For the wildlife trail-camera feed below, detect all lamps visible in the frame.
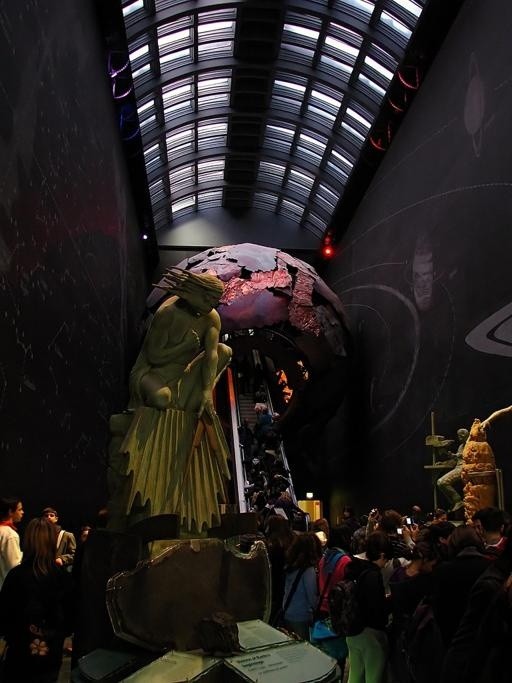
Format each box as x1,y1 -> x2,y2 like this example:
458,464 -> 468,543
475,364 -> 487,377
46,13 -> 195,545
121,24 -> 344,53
321,235 -> 334,257
368,123 -> 391,153
396,65 -> 419,90
107,49 -> 129,79
121,121 -> 139,141
112,80 -> 133,100
387,91 -> 407,114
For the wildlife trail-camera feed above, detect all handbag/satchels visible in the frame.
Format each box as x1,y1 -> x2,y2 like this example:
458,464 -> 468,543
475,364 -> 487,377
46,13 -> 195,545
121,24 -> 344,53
311,614 -> 340,640
272,608 -> 285,626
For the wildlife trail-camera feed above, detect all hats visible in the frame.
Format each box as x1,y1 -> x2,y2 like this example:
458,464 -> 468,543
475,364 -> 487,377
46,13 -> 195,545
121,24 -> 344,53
42,507 -> 57,516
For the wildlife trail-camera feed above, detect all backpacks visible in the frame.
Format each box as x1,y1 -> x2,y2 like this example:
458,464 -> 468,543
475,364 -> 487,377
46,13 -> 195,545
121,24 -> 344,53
328,560 -> 375,636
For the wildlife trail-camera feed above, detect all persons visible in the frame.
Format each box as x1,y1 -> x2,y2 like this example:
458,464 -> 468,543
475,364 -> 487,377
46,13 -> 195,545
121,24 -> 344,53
1,490 -> 133,681
124,264 -> 234,418
434,427 -> 468,516
250,473 -> 512,682
232,367 -> 294,486
